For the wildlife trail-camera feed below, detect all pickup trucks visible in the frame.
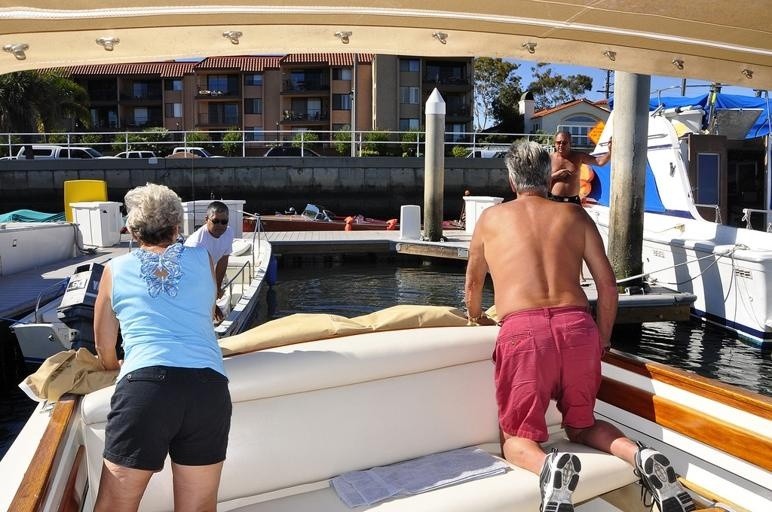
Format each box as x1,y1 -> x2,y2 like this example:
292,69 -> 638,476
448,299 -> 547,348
16,145 -> 121,160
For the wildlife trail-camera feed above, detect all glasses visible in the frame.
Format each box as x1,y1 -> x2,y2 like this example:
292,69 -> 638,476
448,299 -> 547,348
207,218 -> 228,225
554,140 -> 569,145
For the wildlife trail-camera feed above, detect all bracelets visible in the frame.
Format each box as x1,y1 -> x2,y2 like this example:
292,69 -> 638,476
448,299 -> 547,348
467,311 -> 487,323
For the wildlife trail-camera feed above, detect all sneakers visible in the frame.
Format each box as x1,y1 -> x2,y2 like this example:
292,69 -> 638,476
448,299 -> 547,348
539,447 -> 582,512
633,440 -> 696,512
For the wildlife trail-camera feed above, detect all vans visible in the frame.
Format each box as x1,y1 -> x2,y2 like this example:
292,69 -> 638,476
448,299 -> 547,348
173,146 -> 227,158
464,145 -> 511,159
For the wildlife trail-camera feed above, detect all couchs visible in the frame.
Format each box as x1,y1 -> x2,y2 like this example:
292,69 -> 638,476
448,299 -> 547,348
85,323 -> 646,511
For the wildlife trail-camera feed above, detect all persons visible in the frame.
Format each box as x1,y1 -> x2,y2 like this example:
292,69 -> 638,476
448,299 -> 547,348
464,137 -> 700,511
549,130 -> 613,208
182,201 -> 233,321
91,181 -> 233,511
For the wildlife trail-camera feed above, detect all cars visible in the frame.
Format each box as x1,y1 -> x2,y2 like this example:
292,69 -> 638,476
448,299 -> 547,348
264,146 -> 320,157
0,156 -> 17,160
113,150 -> 156,159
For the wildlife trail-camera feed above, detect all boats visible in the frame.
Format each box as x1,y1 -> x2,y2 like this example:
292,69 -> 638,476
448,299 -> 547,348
0,1 -> 772,512
576,77 -> 772,356
12,213 -> 277,367
242,204 -> 465,231
